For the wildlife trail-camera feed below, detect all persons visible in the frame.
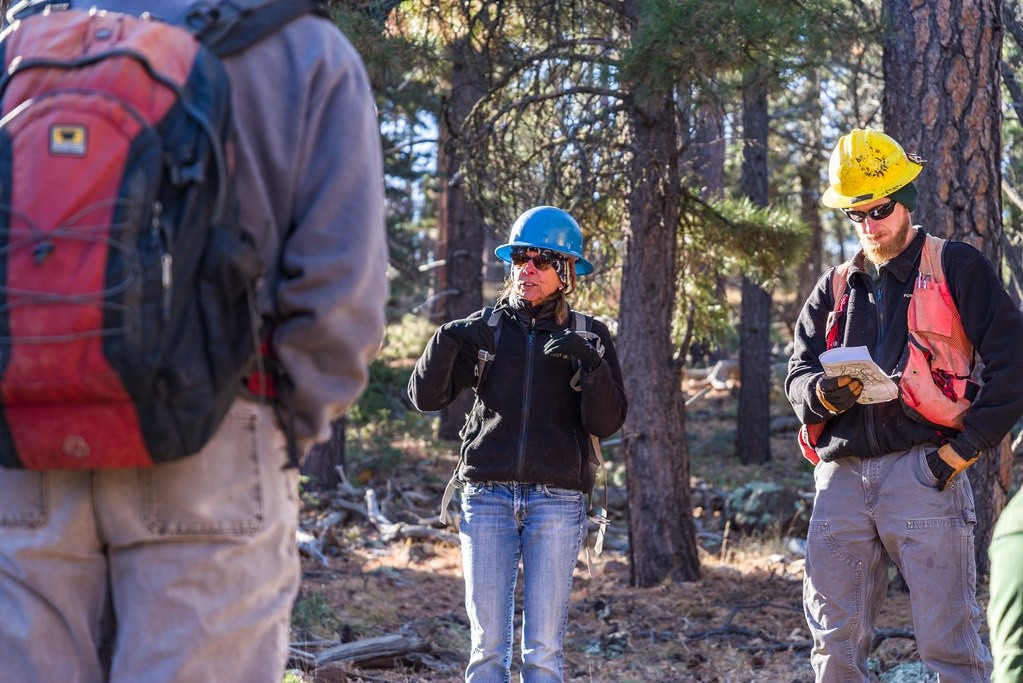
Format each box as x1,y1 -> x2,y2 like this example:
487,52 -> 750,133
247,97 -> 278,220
0,0 -> 383,683
408,206 -> 628,682
787,127 -> 1023,682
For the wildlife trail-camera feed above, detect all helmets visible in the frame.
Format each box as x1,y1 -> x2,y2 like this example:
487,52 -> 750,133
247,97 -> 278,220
822,127 -> 923,208
494,206 -> 593,275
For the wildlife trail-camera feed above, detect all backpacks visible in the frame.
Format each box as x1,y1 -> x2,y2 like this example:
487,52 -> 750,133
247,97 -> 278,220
0,0 -> 337,470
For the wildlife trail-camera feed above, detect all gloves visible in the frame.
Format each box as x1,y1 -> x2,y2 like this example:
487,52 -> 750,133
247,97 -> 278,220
544,327 -> 602,372
926,437 -> 982,492
441,317 -> 496,355
816,372 -> 864,416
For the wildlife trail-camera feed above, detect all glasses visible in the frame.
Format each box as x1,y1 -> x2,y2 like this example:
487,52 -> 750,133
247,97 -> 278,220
846,199 -> 897,222
510,252 -> 568,271
